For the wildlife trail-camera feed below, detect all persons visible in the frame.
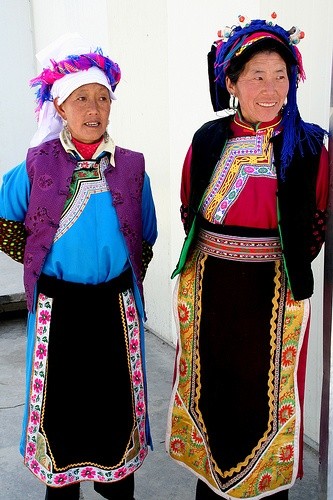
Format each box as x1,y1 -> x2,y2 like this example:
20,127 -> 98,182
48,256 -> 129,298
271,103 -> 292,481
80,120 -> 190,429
180,18 -> 328,500
0,52 -> 158,500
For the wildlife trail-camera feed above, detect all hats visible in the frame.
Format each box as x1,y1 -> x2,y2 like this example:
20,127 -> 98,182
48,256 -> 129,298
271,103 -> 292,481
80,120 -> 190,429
208,12 -> 329,180
29,47 -> 120,147
